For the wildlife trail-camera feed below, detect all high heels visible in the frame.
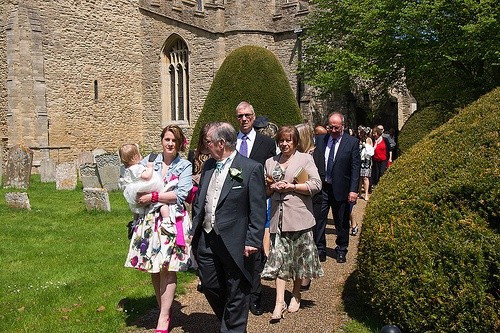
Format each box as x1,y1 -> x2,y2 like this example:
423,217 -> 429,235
154,307 -> 172,333
270,302 -> 288,320
288,299 -> 301,314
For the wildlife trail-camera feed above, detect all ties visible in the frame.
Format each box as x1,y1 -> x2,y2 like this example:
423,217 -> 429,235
239,136 -> 249,158
214,157 -> 232,178
326,138 -> 339,183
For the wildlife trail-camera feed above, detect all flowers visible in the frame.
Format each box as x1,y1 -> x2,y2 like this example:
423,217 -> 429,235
229,167 -> 244,182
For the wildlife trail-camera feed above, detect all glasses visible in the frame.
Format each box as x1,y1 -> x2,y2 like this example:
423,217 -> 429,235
206,140 -> 216,145
237,113 -> 253,119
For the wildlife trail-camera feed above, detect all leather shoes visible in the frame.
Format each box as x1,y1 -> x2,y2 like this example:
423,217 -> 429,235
251,304 -> 263,315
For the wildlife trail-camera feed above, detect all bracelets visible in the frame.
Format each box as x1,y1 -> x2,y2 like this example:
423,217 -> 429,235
293,184 -> 296,192
151,192 -> 158,203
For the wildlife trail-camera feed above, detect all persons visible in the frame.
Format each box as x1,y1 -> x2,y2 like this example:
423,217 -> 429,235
258,122 -> 281,155
295,124 -> 317,155
119,144 -> 177,234
124,124 -> 193,333
314,126 -> 327,134
189,123 -> 268,333
311,112 -> 361,264
343,122 -> 359,235
344,126 -> 396,200
188,122 -> 212,293
264,125 -> 324,320
235,102 -> 276,315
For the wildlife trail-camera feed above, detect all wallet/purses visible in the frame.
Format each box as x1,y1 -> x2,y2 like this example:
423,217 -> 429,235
293,168 -> 310,185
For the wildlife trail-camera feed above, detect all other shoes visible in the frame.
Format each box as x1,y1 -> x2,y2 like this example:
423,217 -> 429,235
337,253 -> 347,263
161,223 -> 177,236
350,223 -> 359,235
300,279 -> 312,291
318,252 -> 327,262
